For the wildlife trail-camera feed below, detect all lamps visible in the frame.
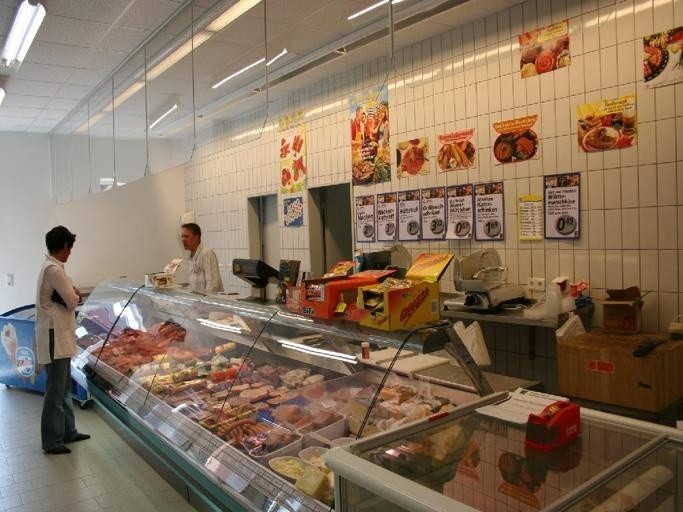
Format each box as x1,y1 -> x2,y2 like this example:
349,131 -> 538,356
0,0 -> 46,72
144,43 -> 290,132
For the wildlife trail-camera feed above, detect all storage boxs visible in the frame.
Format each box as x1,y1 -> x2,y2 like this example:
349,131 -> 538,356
300,273 -> 441,333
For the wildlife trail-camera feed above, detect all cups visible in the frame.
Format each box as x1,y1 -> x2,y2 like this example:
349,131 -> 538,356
623,115 -> 635,136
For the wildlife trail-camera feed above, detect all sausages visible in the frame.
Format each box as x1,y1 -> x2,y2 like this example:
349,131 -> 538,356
216,418 -> 272,448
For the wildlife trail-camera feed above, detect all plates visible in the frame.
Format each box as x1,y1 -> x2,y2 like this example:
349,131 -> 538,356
581,127 -> 620,150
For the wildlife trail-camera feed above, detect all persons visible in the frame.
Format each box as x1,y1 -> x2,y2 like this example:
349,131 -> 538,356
35,224 -> 91,454
180,222 -> 222,294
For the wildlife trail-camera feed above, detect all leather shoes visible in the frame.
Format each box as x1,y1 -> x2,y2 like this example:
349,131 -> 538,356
64,431 -> 92,443
43,445 -> 72,455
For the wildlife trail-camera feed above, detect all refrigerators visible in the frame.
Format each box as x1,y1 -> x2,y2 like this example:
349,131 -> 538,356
0,304 -> 90,408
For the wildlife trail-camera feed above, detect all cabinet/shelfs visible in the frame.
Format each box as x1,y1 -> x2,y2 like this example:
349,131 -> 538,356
0,280 -> 683,512
